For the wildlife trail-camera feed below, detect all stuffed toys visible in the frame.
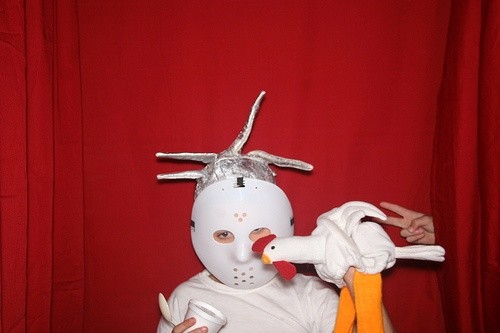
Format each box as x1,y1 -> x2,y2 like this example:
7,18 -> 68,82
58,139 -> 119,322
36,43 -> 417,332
252,201 -> 446,333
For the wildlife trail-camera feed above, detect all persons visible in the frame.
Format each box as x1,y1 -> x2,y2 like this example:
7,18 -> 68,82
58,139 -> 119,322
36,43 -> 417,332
156,91 -> 395,333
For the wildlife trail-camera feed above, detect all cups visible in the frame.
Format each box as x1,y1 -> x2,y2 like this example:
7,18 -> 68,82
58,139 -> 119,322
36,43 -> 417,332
184,299 -> 226,333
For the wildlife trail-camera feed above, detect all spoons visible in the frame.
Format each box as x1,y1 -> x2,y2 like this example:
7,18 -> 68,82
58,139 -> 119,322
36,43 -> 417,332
158,293 -> 179,327
